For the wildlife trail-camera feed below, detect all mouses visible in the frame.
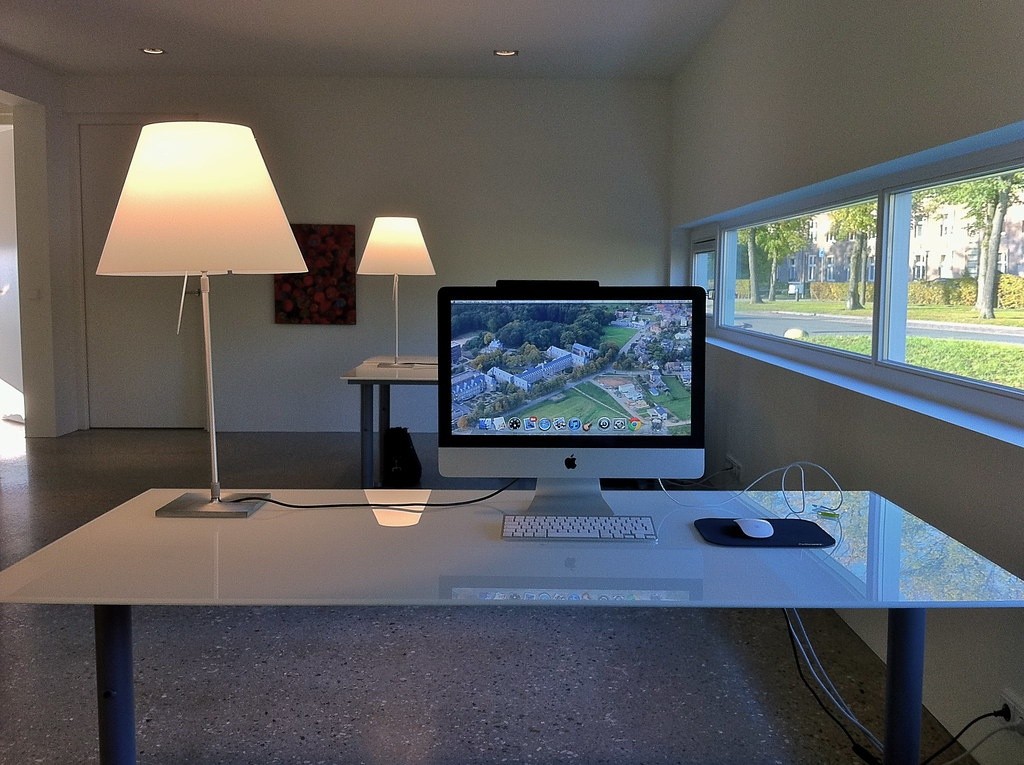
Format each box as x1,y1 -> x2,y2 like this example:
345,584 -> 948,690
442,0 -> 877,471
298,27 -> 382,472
733,519 -> 774,539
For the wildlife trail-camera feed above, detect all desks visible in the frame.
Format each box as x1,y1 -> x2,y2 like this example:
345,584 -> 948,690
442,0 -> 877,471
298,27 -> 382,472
3,478 -> 1024,765
339,353 -> 439,491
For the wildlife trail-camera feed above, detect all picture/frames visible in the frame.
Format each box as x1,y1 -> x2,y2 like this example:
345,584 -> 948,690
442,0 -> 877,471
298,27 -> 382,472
273,220 -> 359,327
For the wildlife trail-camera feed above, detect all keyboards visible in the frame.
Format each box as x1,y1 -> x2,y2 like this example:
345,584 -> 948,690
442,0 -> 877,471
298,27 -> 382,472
501,514 -> 658,543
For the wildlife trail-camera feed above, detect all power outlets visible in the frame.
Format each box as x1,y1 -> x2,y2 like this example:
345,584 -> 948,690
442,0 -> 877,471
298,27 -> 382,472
997,688 -> 1024,737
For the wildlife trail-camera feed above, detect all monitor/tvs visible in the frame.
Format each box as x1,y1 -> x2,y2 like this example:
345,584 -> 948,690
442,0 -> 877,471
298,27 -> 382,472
436,286 -> 707,476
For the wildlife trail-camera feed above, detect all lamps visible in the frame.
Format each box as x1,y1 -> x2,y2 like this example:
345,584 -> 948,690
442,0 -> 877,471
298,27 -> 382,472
355,214 -> 438,371
88,113 -> 313,520
